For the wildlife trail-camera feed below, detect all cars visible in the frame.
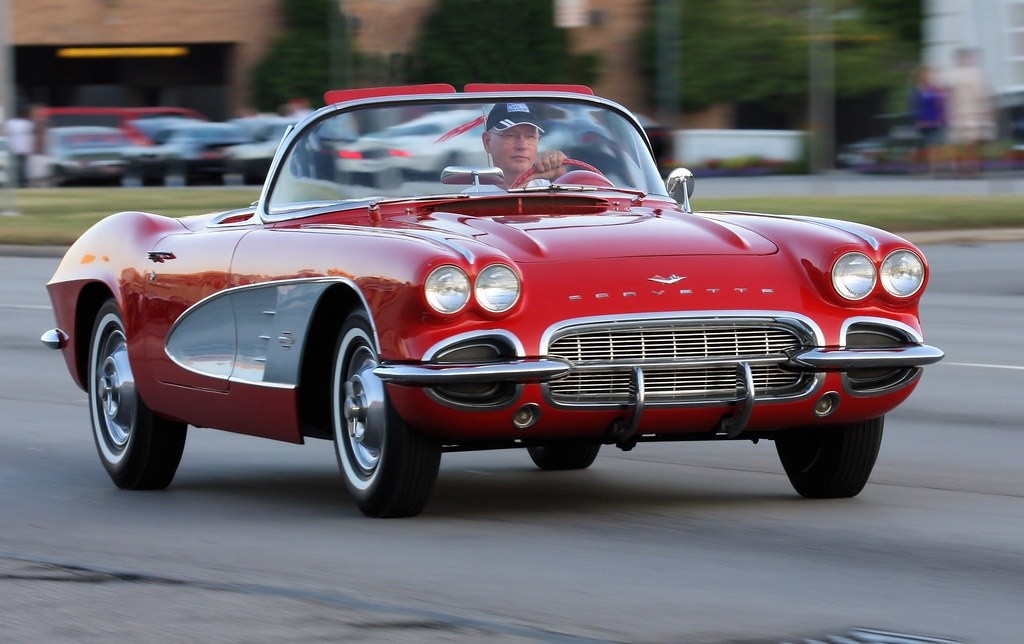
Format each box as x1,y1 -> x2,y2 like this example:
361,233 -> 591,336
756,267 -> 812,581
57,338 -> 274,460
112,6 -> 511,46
0,113 -> 671,189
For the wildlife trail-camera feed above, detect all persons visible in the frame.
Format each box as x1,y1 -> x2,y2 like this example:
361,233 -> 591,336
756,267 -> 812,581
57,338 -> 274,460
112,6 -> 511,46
3,112 -> 35,187
461,102 -> 569,193
912,46 -> 997,147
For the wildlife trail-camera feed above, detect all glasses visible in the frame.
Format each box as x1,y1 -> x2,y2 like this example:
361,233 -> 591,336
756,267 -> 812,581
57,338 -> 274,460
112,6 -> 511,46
487,130 -> 542,145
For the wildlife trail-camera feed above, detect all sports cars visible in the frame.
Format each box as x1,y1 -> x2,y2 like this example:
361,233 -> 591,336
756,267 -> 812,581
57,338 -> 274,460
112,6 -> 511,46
40,83 -> 945,520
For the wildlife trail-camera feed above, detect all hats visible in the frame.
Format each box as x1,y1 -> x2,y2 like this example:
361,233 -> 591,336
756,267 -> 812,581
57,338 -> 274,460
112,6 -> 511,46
486,102 -> 546,134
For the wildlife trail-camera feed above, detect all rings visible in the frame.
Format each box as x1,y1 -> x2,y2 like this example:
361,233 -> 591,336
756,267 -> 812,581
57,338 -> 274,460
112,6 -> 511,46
550,154 -> 557,158
